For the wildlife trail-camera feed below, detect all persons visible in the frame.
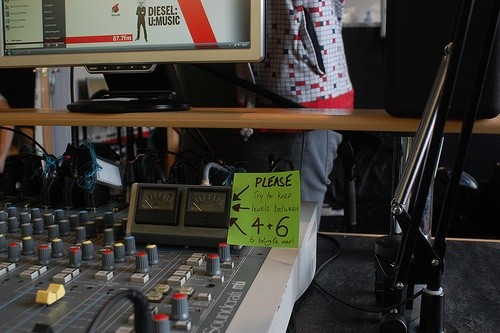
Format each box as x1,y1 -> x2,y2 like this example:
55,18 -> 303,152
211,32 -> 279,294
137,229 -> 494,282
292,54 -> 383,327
249,0 -> 354,231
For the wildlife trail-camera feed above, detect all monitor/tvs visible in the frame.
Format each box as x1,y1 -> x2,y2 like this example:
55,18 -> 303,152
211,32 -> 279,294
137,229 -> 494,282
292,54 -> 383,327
0,0 -> 268,114
